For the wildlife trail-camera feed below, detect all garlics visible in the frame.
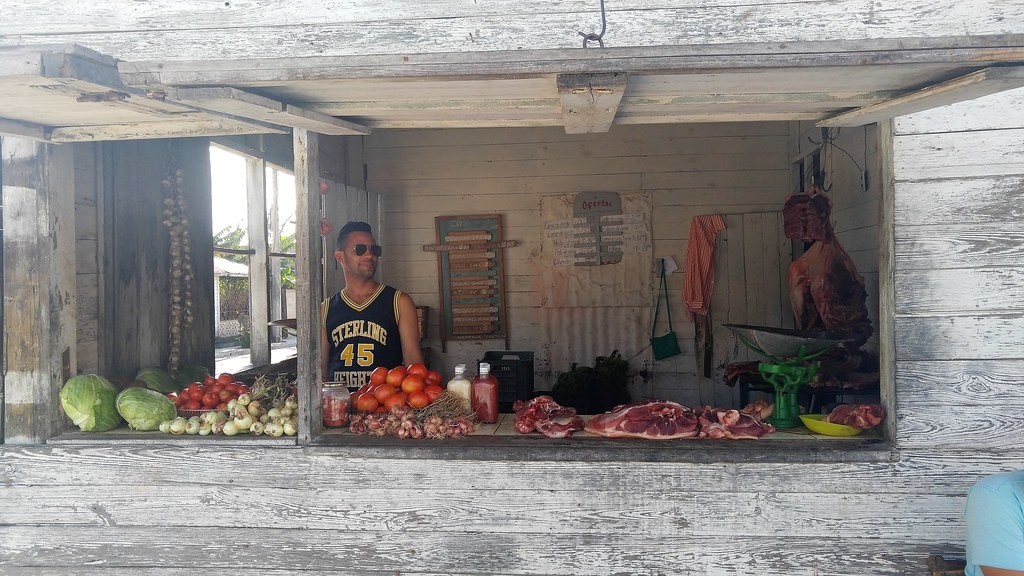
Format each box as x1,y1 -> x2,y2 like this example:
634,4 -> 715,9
161,169 -> 193,373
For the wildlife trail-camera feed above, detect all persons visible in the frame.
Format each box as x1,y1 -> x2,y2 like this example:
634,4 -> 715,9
320,221 -> 423,393
963,468 -> 1024,576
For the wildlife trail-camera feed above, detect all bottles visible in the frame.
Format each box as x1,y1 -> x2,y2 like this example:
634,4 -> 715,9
473,363 -> 498,424
448,364 -> 473,419
323,382 -> 350,429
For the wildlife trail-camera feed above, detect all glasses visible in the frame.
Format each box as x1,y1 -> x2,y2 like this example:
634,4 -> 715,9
339,244 -> 382,257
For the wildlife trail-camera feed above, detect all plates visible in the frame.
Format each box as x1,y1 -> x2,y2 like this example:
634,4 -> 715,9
800,414 -> 864,437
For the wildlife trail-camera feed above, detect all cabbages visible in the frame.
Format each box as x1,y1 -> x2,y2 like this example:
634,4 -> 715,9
59,364 -> 176,432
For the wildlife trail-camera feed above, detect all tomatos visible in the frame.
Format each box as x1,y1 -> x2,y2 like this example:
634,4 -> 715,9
166,372 -> 250,416
349,363 -> 442,413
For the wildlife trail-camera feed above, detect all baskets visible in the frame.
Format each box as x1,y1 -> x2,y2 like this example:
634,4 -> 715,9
175,408 -> 229,418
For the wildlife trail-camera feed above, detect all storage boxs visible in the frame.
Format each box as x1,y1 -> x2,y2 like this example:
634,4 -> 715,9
477,351 -> 535,412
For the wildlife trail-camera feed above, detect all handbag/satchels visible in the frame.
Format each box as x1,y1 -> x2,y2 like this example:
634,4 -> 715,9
649,331 -> 682,362
594,349 -> 628,384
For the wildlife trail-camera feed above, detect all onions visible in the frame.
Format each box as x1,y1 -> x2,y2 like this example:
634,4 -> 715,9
159,394 -> 299,438
346,405 -> 477,440
